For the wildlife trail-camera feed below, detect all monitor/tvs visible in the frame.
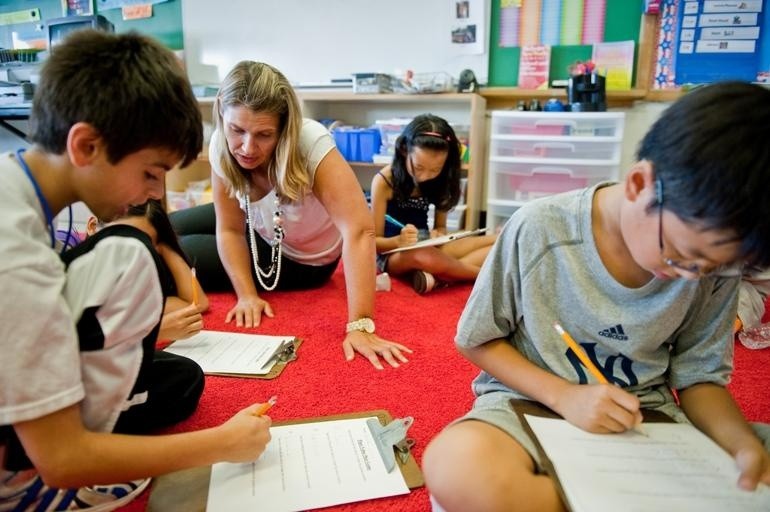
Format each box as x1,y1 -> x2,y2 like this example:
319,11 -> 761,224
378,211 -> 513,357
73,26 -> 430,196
47,14 -> 115,47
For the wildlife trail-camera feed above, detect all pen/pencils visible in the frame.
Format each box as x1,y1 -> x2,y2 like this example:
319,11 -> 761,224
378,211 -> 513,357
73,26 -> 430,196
551,319 -> 650,437
569,61 -> 599,103
384,214 -> 424,241
191,267 -> 198,308
254,396 -> 278,418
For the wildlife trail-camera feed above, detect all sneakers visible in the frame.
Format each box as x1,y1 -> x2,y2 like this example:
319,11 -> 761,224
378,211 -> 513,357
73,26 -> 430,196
0,474 -> 154,512
406,269 -> 437,294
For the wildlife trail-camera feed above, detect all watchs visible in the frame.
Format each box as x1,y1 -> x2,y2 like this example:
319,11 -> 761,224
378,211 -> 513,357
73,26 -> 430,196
345,316 -> 376,334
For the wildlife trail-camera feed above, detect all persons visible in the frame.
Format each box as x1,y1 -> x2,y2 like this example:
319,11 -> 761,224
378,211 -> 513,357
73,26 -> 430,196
166,60 -> 414,371
56,194 -> 212,345
0,25 -> 274,510
367,111 -> 497,298
414,79 -> 770,512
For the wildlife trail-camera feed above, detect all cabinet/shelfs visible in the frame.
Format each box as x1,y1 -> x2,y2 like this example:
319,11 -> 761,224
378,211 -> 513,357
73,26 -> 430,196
165,96 -> 217,213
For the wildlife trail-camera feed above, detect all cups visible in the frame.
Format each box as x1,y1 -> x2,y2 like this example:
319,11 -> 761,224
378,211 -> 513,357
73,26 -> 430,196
738,323 -> 770,350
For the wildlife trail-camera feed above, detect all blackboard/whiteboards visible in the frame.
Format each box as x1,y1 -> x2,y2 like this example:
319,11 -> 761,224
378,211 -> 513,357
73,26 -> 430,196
181,0 -> 492,88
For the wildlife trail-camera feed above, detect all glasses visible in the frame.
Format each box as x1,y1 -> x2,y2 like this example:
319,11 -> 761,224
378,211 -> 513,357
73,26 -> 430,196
641,156 -> 750,283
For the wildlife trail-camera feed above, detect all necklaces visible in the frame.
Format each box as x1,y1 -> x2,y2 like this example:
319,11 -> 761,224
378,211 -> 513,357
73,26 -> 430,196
243,186 -> 286,293
